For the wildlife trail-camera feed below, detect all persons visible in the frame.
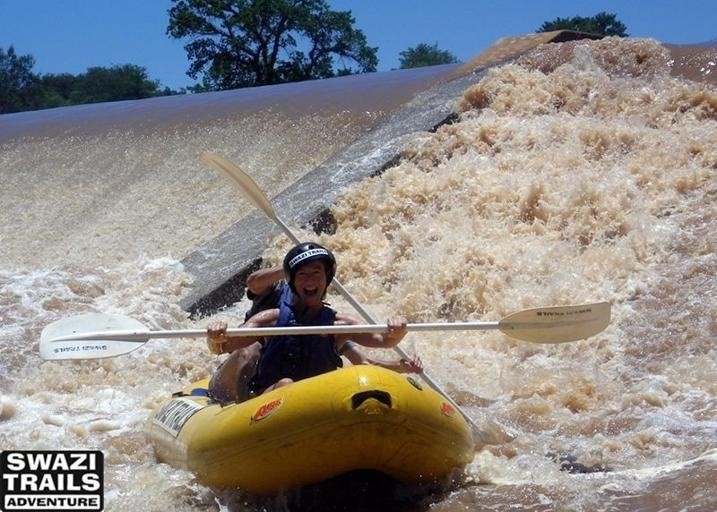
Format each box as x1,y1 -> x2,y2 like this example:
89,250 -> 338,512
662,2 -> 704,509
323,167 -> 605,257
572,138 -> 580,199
209,264 -> 424,402
206,242 -> 407,395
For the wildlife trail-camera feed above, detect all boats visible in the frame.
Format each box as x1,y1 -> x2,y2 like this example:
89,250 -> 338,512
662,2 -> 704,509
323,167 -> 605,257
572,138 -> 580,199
143,365 -> 478,496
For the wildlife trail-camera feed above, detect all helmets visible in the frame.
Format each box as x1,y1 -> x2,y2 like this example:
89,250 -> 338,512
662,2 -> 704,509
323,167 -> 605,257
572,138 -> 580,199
282,241 -> 337,293
